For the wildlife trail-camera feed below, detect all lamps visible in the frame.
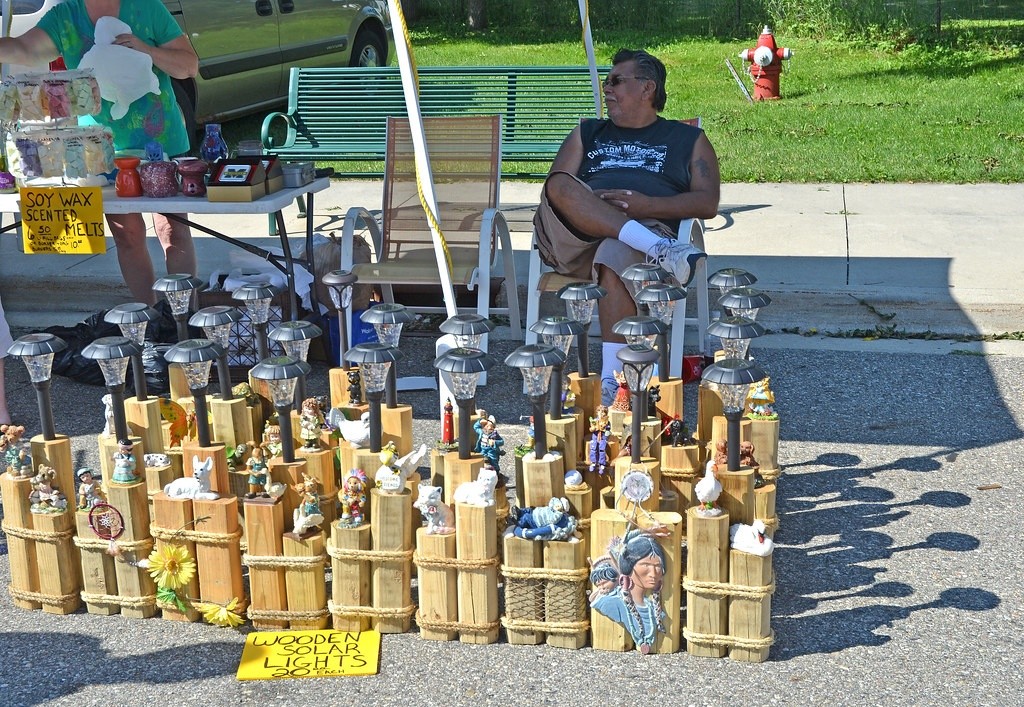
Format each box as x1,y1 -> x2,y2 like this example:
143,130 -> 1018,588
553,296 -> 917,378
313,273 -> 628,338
560,282 -> 607,376
616,344 -> 660,463
233,283 -> 280,361
718,288 -> 771,322
343,341 -> 402,453
267,321 -> 323,417
250,356 -> 311,463
635,284 -> 689,380
323,269 -> 359,371
707,268 -> 758,298
8,273 -> 243,447
702,358 -> 764,471
432,349 -> 498,460
503,344 -> 565,457
438,313 -> 495,418
622,264 -> 673,316
526,318 -> 587,417
706,316 -> 764,359
612,316 -> 669,419
359,305 -> 415,408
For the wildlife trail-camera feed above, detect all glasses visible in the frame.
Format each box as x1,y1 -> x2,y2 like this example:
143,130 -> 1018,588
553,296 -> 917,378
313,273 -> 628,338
602,75 -> 649,88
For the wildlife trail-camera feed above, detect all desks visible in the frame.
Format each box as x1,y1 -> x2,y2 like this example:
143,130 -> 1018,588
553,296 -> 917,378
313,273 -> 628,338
0,160 -> 328,363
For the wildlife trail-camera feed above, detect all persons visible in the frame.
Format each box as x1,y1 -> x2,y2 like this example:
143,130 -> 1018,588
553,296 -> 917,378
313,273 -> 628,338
78,466 -> 103,509
112,438 -> 139,481
262,426 -> 284,457
561,376 -> 576,414
512,498 -> 571,539
0,0 -> 202,308
587,407 -> 615,474
532,48 -> 721,405
300,397 -> 322,448
473,414 -> 506,476
525,418 -> 538,448
0,302 -> 13,433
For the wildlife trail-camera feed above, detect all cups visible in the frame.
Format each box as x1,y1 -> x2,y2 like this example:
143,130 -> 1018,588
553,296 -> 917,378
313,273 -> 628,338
237,141 -> 263,156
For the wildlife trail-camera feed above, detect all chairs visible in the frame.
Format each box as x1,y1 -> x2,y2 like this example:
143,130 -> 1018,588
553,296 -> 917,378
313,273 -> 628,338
339,116 -> 522,392
523,115 -> 702,394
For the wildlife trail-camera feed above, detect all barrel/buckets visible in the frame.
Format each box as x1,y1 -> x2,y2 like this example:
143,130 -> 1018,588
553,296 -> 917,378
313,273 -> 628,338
326,300 -> 381,366
683,355 -> 704,383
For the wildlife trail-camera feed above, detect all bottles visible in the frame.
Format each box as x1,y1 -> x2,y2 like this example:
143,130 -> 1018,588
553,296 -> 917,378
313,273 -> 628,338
113,158 -> 142,197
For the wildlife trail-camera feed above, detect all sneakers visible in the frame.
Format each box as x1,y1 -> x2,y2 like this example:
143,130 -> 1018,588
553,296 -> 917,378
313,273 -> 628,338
601,376 -> 618,407
646,237 -> 707,287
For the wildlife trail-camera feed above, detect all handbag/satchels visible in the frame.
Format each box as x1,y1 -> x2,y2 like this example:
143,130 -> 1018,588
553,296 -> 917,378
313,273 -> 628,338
324,301 -> 383,366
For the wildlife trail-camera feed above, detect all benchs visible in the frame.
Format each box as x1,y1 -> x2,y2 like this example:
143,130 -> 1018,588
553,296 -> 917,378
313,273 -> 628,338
262,67 -> 610,237
